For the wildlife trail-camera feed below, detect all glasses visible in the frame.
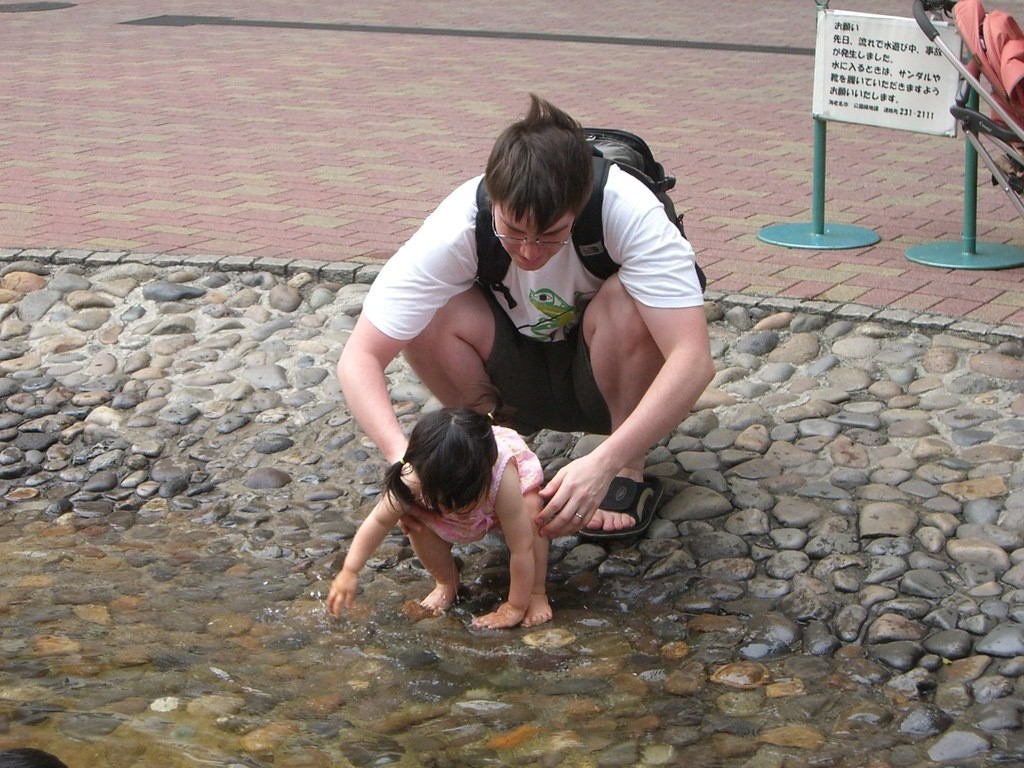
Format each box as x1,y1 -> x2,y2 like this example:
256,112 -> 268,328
492,204 -> 575,247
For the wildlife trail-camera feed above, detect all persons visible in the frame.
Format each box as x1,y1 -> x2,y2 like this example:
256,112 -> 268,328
325,379 -> 553,629
337,90 -> 716,538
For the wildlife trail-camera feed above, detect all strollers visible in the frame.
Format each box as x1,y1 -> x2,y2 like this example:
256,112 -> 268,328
911,0 -> 1024,218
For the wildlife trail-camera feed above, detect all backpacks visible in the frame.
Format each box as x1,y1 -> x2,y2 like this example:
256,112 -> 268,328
476,128 -> 707,310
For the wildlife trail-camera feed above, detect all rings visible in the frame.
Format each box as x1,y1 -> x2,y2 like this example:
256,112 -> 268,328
575,513 -> 583,519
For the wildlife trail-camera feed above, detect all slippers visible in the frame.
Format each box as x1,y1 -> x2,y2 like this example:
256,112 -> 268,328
578,474 -> 664,540
